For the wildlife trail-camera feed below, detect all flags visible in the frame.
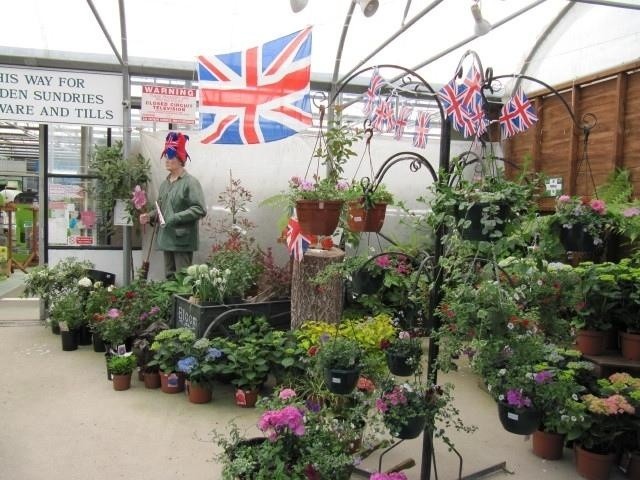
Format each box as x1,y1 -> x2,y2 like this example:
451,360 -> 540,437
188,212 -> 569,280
283,217 -> 314,264
193,24 -> 315,145
359,65 -> 540,151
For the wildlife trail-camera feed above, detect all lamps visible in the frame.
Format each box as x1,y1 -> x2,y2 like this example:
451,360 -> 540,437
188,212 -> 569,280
290,0 -> 308,13
352,0 -> 379,17
471,4 -> 491,36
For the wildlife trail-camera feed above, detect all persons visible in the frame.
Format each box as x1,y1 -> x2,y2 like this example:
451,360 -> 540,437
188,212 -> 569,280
139,131 -> 208,281
3,229 -> 9,244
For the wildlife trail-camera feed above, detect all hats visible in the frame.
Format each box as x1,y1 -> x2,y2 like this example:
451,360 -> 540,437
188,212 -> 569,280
161,133 -> 190,162
